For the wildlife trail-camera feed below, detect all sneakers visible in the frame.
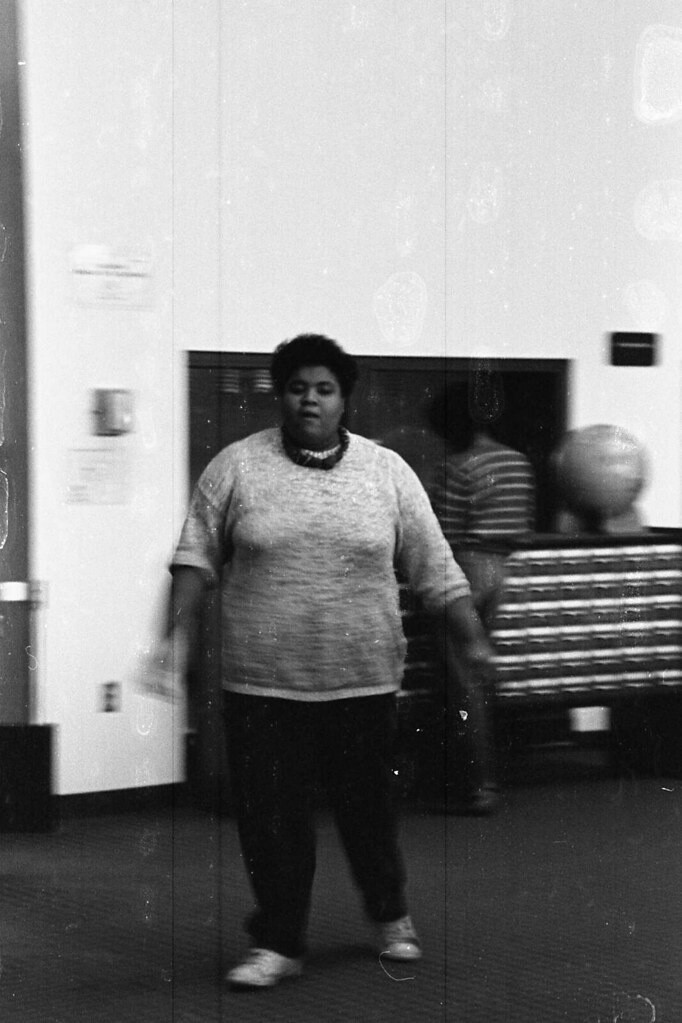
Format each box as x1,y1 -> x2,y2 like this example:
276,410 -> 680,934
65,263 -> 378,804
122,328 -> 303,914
225,948 -> 304,986
368,915 -> 422,982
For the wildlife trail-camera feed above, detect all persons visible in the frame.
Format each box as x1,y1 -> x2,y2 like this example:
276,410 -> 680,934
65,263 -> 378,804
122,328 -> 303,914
428,391 -> 540,816
144,334 -> 496,990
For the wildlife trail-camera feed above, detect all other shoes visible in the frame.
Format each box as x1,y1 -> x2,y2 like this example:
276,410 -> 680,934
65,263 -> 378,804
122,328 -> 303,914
430,787 -> 500,813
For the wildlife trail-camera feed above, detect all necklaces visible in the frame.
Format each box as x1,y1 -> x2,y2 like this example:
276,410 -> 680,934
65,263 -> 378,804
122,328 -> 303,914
281,422 -> 350,470
